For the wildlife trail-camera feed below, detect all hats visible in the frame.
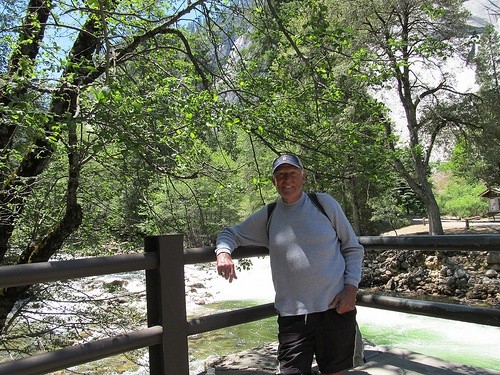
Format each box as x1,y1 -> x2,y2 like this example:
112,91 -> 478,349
272,154 -> 304,172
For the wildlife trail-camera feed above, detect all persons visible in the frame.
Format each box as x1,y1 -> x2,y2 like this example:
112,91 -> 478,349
214,155 -> 364,375
